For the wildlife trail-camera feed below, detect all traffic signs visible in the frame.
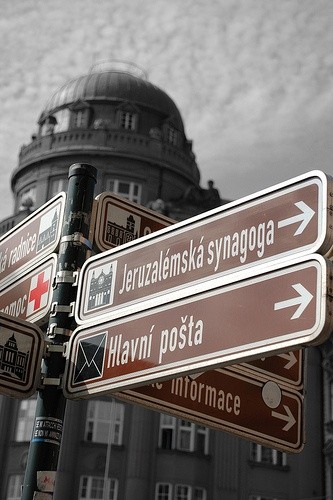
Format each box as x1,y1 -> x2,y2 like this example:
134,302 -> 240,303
73,170 -> 333,333
0,252 -> 59,326
108,361 -> 306,456
0,311 -> 46,403
0,190 -> 67,292
61,251 -> 331,407
220,340 -> 306,391
88,191 -> 180,255
